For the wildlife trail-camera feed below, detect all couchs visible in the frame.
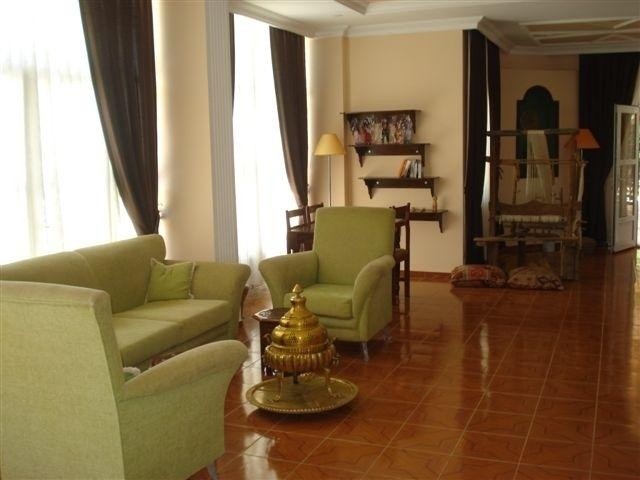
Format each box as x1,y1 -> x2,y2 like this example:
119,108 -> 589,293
0,231 -> 252,365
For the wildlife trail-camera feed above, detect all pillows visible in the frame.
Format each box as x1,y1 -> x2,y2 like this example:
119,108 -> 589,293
144,256 -> 196,301
449,264 -> 564,292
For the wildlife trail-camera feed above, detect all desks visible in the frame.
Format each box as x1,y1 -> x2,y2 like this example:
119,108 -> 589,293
252,307 -> 307,383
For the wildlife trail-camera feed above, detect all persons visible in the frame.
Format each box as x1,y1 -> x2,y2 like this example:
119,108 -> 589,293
350,113 -> 413,145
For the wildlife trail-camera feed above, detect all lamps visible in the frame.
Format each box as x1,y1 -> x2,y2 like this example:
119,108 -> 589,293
566,129 -> 602,164
312,132 -> 346,209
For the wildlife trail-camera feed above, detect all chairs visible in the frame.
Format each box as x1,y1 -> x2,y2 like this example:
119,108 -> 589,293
256,205 -> 398,363
286,202 -> 412,305
0,281 -> 250,480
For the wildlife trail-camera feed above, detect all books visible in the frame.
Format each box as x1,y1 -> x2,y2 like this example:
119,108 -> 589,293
400,159 -> 422,179
